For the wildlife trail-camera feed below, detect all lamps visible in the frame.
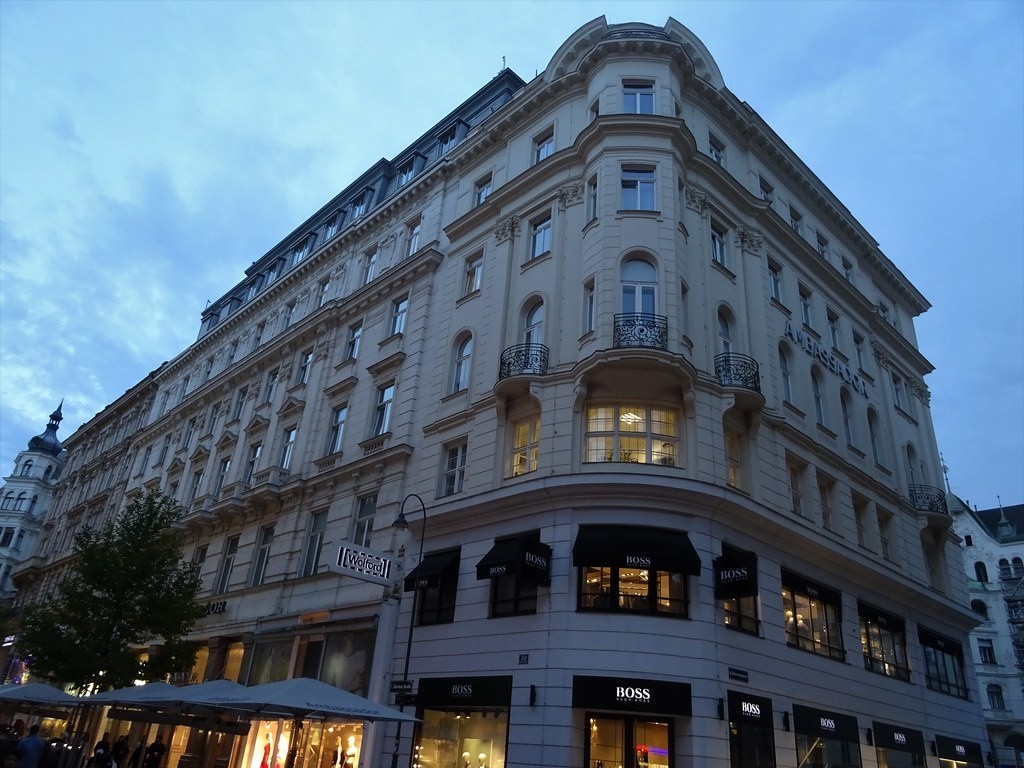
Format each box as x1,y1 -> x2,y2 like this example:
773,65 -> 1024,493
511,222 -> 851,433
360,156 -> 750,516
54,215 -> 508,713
619,407 -> 641,424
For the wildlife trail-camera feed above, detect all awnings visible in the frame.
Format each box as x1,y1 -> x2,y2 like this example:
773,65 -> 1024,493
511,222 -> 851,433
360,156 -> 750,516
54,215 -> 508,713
476,538 -> 550,579
404,547 -> 460,591
572,524 -> 701,576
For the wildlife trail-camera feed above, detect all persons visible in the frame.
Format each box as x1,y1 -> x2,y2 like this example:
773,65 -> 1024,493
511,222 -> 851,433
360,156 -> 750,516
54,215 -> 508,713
259,733 -> 284,768
327,637 -> 367,697
259,647 -> 286,684
331,736 -> 358,768
0,724 -> 166,768
786,610 -> 809,649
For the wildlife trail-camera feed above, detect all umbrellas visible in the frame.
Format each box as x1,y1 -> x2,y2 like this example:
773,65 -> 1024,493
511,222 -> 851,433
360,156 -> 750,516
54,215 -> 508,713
0,677 -> 426,723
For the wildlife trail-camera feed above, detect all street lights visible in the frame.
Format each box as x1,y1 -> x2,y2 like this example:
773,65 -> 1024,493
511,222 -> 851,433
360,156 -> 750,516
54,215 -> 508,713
389,493 -> 427,768
